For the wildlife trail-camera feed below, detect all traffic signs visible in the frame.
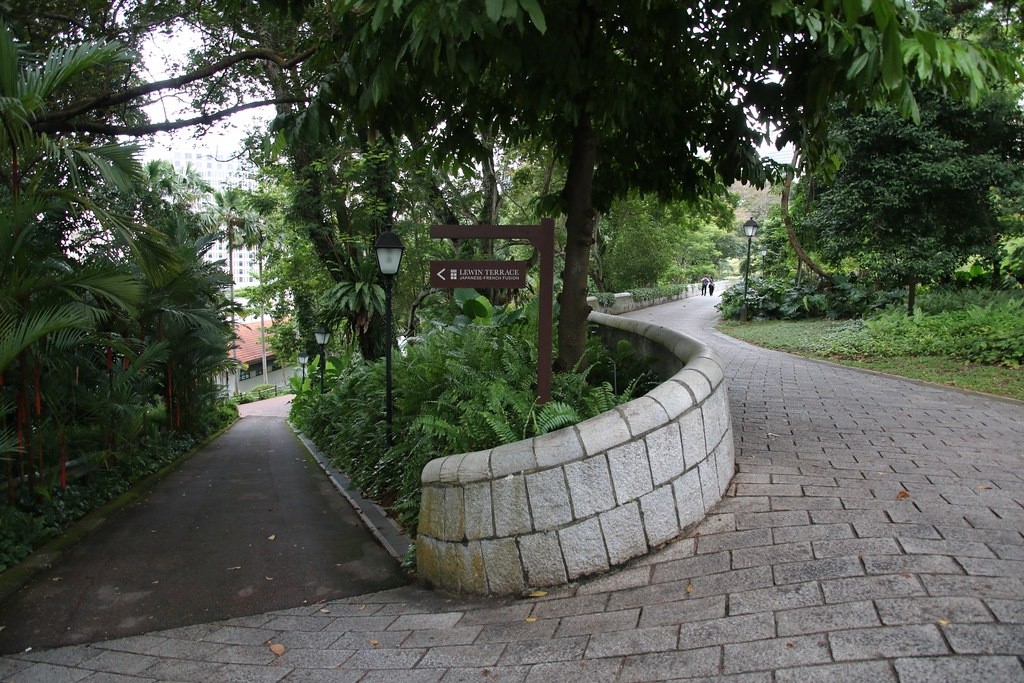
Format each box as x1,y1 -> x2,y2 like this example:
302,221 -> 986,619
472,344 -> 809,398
428,258 -> 527,290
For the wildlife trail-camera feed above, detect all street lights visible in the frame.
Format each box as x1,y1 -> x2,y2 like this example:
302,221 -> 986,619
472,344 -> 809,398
740,217 -> 758,323
761,244 -> 768,278
372,222 -> 407,453
312,323 -> 331,394
297,350 -> 310,385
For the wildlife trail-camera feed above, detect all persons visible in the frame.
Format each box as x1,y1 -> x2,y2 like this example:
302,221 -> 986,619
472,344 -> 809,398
700,276 -> 709,296
708,277 -> 715,296
